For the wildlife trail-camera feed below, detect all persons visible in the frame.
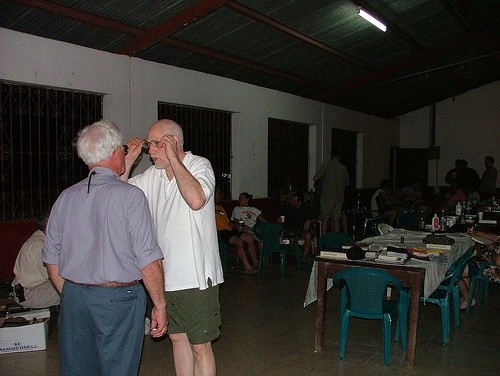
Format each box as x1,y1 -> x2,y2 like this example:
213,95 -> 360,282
214,188 -> 259,274
445,156 -> 497,204
41,120 -> 168,376
276,193 -> 320,264
371,179 -> 417,226
313,149 -> 350,235
11,216 -> 61,337
447,228 -> 500,309
231,192 -> 274,266
119,119 -> 224,376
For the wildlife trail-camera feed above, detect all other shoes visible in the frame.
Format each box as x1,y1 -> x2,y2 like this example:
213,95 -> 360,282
144,318 -> 152,336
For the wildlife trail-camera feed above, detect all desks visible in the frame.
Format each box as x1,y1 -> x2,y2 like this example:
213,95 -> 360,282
312,228 -> 481,369
409,201 -> 500,236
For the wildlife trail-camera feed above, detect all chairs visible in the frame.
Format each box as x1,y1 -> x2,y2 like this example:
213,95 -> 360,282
216,209 -> 500,366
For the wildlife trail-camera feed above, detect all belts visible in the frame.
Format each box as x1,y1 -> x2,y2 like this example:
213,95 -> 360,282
74,280 -> 139,287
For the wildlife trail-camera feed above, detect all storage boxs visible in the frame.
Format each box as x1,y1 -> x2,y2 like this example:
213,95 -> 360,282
0,318 -> 50,355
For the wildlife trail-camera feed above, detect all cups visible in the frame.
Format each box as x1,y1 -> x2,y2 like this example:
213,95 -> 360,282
281,216 -> 285,222
478,212 -> 483,221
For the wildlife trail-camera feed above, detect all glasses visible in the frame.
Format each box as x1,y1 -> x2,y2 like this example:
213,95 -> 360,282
144,140 -> 160,148
113,145 -> 129,156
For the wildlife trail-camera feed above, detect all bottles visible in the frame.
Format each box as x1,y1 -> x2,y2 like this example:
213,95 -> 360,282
449,218 -> 454,228
432,214 -> 439,231
462,202 -> 466,209
441,210 -> 447,231
461,216 -> 465,226
456,202 -> 461,215
419,210 -> 425,230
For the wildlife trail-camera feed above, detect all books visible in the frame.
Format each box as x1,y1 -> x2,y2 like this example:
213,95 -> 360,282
342,243 -> 369,250
426,234 -> 451,251
379,245 -> 409,264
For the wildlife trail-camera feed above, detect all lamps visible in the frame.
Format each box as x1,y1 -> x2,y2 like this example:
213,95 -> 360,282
355,6 -> 388,32
